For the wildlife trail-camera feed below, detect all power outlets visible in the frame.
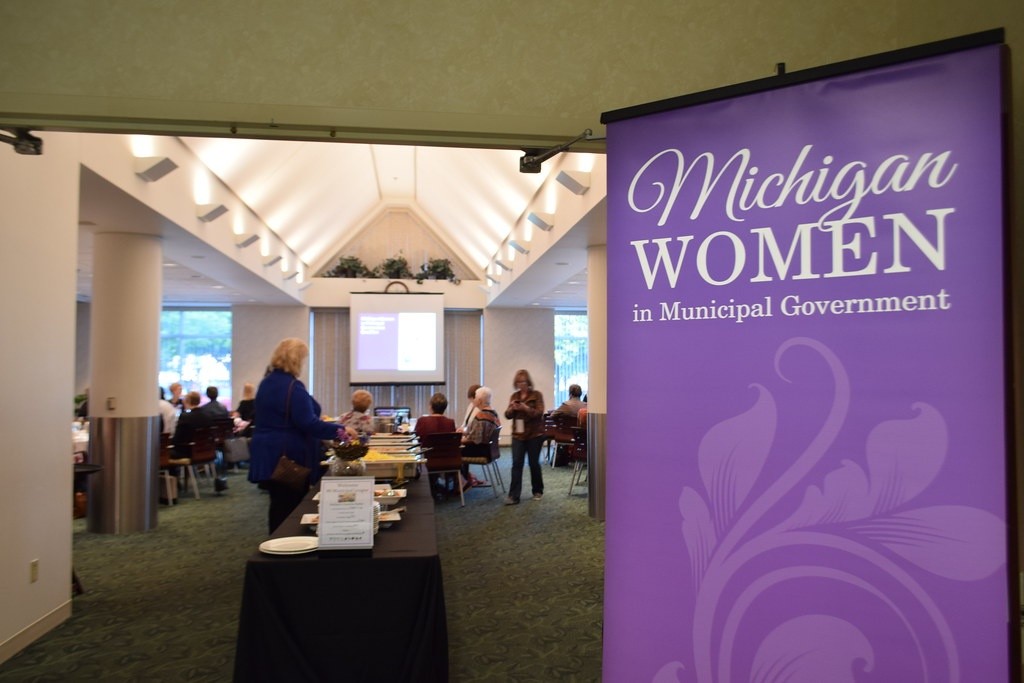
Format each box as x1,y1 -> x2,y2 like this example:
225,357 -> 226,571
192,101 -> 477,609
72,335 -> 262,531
29,559 -> 39,584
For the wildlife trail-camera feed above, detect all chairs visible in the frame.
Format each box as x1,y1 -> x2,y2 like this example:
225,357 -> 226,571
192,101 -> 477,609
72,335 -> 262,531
422,432 -> 465,507
462,426 -> 506,499
158,417 -> 234,506
545,409 -> 587,498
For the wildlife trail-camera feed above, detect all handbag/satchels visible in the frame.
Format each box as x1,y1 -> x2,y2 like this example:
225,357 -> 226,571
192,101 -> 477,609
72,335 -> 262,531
215,477 -> 229,491
271,456 -> 311,491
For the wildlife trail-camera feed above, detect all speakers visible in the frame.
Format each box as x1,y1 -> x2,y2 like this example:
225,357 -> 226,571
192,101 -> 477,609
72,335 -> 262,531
283,271 -> 299,280
261,255 -> 282,266
235,233 -> 260,248
197,203 -> 228,223
135,156 -> 178,183
487,273 -> 500,283
299,283 -> 314,290
479,284 -> 490,294
556,169 -> 591,195
495,259 -> 513,271
509,240 -> 530,254
528,211 -> 554,231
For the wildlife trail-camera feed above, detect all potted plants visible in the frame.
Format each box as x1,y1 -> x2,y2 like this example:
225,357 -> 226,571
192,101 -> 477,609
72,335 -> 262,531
416,257 -> 462,286
323,254 -> 374,278
371,249 -> 416,278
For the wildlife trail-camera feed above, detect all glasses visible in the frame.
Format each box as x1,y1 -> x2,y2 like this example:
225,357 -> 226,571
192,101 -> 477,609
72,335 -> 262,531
517,382 -> 526,385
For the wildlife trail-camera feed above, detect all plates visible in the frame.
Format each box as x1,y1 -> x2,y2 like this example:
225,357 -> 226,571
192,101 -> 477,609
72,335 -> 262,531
259,536 -> 320,554
300,512 -> 401,530
312,489 -> 408,504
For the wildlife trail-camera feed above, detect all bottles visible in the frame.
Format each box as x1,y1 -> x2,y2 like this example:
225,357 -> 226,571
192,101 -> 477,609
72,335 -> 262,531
391,411 -> 410,433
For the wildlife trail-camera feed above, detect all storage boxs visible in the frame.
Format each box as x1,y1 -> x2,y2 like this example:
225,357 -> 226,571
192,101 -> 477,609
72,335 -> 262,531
369,432 -> 416,438
368,446 -> 422,454
366,438 -> 420,446
322,454 -> 427,477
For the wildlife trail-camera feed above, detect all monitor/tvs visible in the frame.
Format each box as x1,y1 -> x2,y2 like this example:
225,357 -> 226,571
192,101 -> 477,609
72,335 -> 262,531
373,407 -> 410,431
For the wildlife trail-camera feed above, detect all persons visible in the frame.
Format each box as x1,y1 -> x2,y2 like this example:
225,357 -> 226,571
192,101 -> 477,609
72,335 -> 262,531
246,337 -> 359,536
455,384 -> 501,495
550,384 -> 587,427
229,382 -> 256,438
414,392 -> 457,502
159,383 -> 229,460
503,369 -> 544,505
335,389 -> 376,433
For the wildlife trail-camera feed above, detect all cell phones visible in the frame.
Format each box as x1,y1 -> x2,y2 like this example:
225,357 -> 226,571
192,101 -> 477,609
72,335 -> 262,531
514,400 -> 520,404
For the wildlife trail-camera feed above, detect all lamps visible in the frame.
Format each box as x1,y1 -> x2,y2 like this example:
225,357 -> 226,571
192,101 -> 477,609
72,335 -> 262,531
479,285 -> 491,294
196,203 -> 229,223
134,155 -> 178,183
555,169 -> 592,196
235,234 -> 260,248
298,282 -> 312,291
263,255 -> 283,267
508,239 -> 531,256
527,211 -> 554,231
485,274 -> 501,284
495,259 -> 513,272
282,271 -> 300,280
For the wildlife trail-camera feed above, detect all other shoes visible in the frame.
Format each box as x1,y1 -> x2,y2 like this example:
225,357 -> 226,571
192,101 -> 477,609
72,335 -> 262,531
532,493 -> 544,500
451,489 -> 459,495
505,498 -> 517,504
464,476 -> 483,492
436,493 -> 442,501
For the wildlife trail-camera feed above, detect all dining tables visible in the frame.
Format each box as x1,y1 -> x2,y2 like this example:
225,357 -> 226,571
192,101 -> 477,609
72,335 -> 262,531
237,431 -> 449,683
